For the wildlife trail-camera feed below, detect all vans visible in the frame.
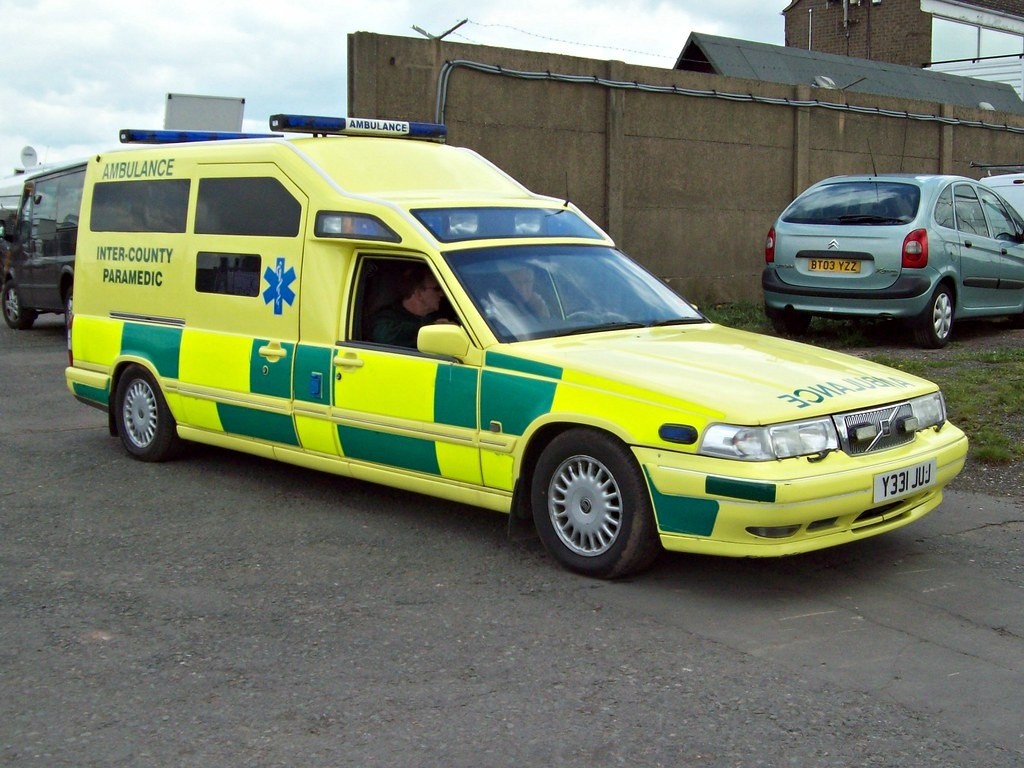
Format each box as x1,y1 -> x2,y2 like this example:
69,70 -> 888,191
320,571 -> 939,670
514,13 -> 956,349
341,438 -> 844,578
0,159 -> 89,332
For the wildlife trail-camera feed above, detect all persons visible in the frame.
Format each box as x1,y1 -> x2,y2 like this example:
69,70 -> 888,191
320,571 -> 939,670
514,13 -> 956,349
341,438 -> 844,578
0,214 -> 18,243
369,267 -> 459,353
488,260 -> 554,342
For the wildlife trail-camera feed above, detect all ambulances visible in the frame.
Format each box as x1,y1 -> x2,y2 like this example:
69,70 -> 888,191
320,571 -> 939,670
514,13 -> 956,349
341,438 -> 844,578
64,112 -> 970,581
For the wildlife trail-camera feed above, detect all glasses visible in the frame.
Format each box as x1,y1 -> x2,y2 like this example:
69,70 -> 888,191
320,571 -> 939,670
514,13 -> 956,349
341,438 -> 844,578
420,285 -> 443,292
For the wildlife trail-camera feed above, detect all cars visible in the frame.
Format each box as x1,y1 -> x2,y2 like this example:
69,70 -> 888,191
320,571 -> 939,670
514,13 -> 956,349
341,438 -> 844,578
761,173 -> 1024,349
978,173 -> 1023,229
